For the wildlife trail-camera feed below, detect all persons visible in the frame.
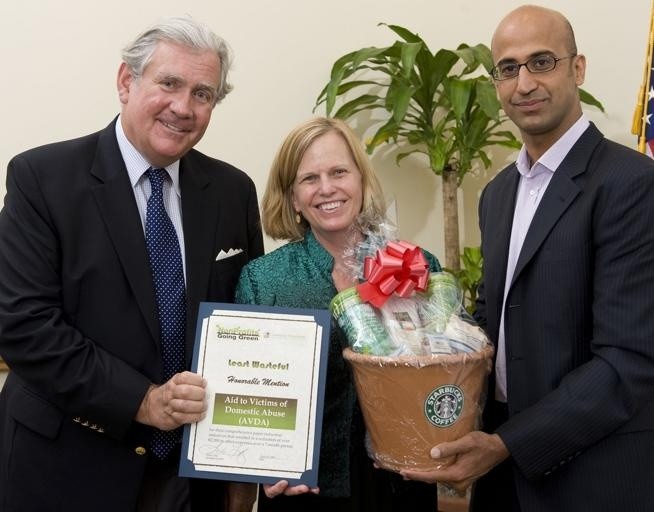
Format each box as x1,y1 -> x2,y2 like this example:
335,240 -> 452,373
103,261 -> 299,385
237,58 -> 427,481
0,17 -> 266,512
235,117 -> 442,512
401,2 -> 654,512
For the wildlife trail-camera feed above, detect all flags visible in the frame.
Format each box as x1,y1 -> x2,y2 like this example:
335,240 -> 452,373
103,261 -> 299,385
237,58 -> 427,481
632,6 -> 654,158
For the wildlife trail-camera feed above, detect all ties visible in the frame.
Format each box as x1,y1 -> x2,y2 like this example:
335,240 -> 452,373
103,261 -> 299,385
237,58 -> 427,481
146,167 -> 186,461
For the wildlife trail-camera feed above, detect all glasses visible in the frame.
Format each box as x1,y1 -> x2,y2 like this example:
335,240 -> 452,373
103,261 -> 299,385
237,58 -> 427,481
489,55 -> 574,82
